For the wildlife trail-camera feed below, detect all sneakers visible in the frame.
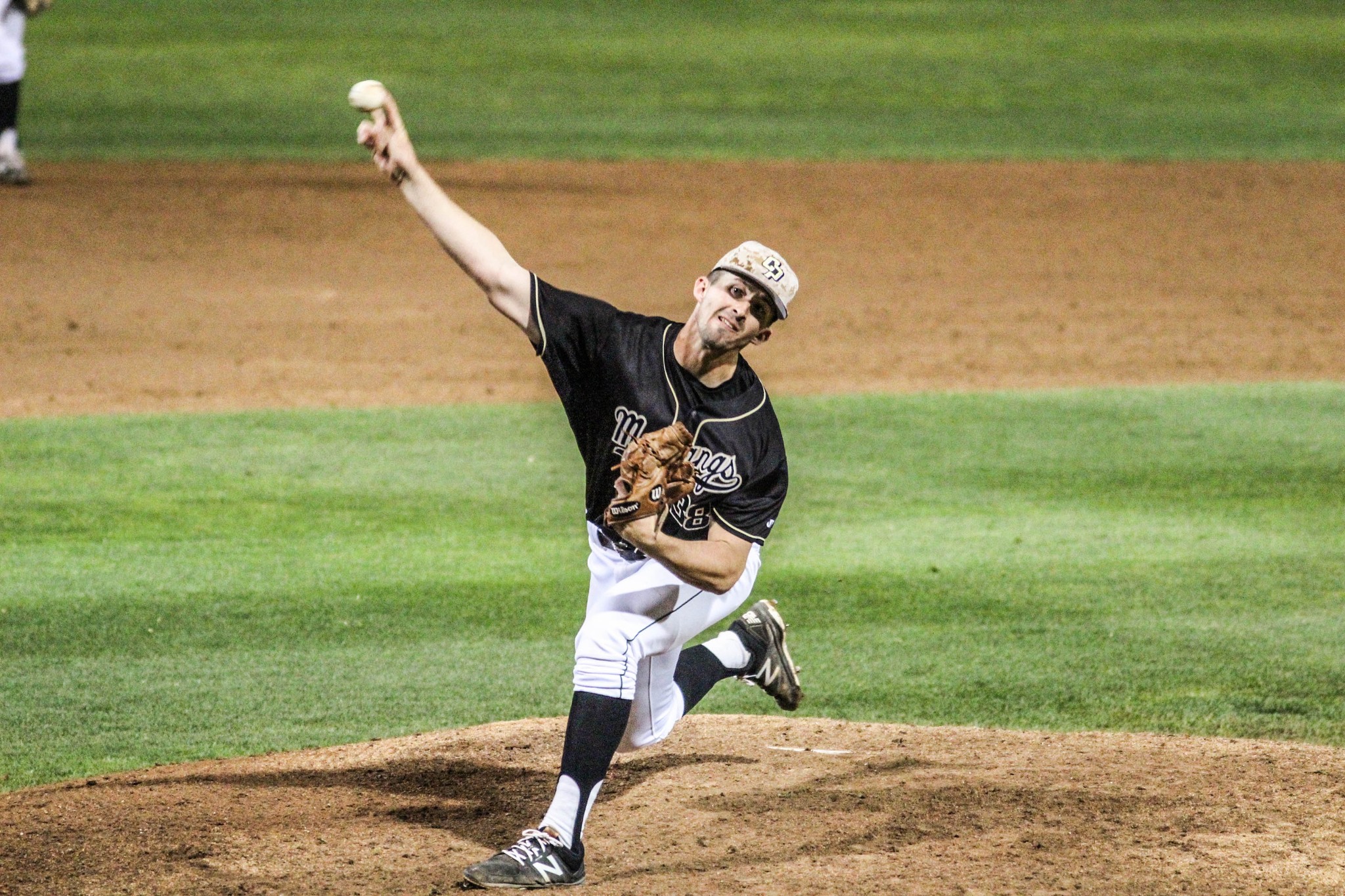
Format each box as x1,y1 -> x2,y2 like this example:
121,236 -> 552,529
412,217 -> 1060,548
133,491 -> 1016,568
732,599 -> 803,710
464,826 -> 587,890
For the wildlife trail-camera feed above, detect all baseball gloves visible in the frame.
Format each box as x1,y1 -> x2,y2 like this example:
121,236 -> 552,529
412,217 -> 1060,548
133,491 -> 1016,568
602,421 -> 698,529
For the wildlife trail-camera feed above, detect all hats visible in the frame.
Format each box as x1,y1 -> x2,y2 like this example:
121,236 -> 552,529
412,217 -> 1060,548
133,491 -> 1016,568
711,239 -> 798,321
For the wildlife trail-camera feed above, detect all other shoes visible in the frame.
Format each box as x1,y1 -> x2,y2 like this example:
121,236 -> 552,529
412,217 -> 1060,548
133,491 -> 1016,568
0,150 -> 29,186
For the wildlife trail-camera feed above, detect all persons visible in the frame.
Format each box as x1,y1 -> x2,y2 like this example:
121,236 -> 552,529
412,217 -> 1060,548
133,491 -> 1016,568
0,1 -> 55,188
345,92 -> 808,892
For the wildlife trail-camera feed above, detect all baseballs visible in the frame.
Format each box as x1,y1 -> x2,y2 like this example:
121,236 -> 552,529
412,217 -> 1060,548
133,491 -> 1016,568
347,78 -> 387,113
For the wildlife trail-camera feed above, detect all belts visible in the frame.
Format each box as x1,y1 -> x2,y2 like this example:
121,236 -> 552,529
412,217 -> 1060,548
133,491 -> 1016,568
598,531 -> 648,562
